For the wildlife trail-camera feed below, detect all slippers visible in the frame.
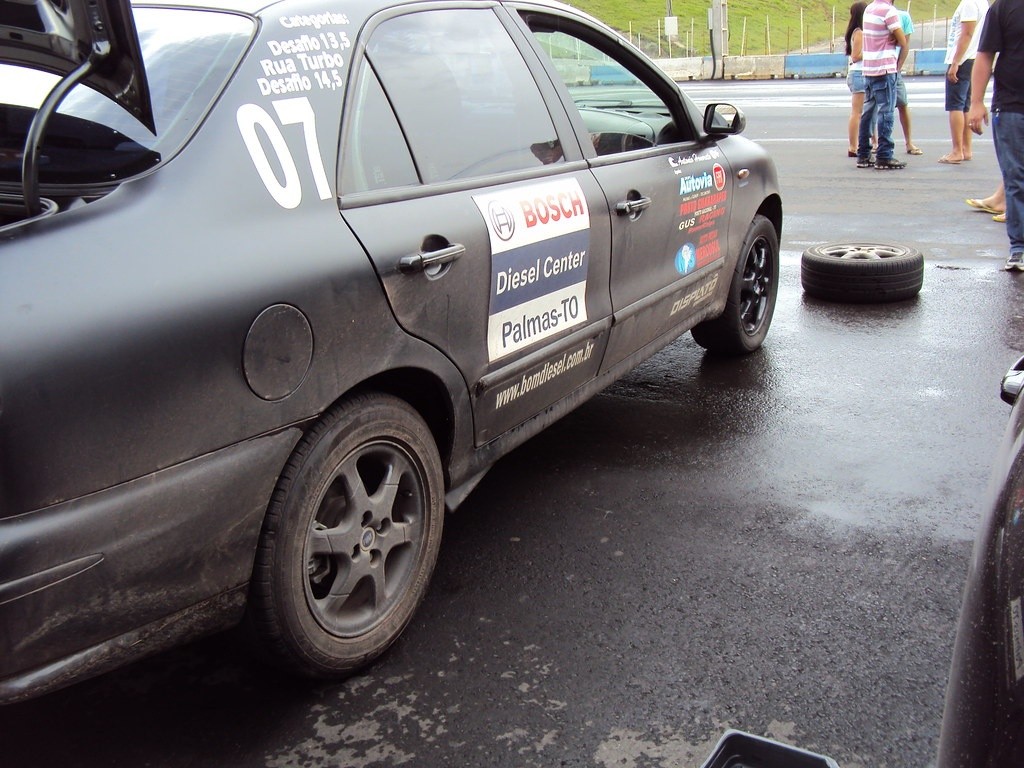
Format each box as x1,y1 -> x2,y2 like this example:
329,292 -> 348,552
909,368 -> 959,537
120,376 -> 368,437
966,199 -> 1003,215
938,153 -> 961,164
907,148 -> 924,155
964,156 -> 973,161
991,215 -> 1006,222
871,145 -> 877,153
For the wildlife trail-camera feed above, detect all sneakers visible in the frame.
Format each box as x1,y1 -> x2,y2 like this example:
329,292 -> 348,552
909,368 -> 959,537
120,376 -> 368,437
874,158 -> 908,169
857,155 -> 876,166
1005,251 -> 1024,271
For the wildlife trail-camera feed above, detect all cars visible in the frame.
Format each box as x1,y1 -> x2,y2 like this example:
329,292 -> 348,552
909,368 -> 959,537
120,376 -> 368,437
1,0 -> 784,685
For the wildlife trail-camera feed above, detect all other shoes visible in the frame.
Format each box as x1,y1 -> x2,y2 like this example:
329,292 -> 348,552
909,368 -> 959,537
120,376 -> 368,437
848,149 -> 857,156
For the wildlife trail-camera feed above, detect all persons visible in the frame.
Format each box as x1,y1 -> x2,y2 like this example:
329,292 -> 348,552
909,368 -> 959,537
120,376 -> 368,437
966,177 -> 1006,222
937,0 -> 990,165
969,0 -> 1024,272
844,0 -> 923,170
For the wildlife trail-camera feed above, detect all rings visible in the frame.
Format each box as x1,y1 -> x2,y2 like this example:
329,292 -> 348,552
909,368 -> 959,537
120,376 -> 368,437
969,123 -> 972,126
